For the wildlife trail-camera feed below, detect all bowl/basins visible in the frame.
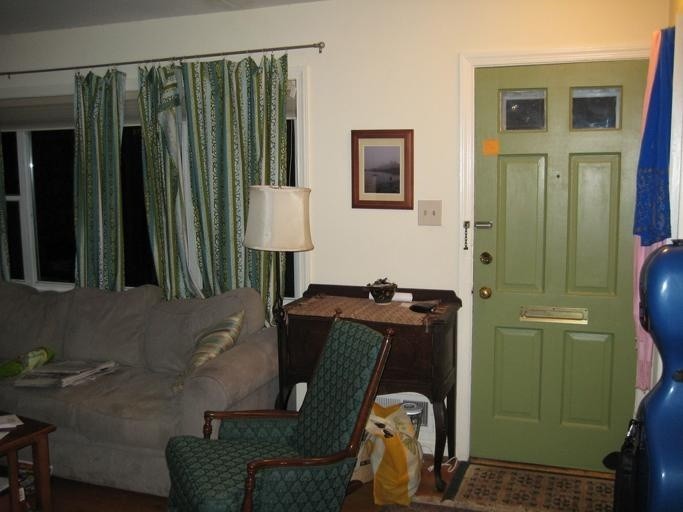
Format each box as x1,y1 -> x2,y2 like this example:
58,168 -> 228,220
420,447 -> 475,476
367,283 -> 397,306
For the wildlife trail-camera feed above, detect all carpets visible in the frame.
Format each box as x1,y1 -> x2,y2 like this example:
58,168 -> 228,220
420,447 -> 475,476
441,460 -> 615,512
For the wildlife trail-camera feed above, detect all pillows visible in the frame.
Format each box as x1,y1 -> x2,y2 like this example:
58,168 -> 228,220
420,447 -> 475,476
170,309 -> 244,392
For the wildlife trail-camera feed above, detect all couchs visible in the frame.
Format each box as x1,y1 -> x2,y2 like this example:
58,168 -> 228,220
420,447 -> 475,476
1,279 -> 279,499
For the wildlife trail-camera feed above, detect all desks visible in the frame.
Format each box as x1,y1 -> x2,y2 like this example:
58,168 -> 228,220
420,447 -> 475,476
0,410 -> 56,512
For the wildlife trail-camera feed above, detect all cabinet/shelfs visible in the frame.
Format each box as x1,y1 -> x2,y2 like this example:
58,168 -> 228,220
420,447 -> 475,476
274,284 -> 462,492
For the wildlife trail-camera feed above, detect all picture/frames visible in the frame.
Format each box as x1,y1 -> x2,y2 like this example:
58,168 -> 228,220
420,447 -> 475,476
352,130 -> 413,209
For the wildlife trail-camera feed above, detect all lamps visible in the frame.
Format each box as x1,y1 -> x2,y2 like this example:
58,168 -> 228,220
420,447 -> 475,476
242,180 -> 314,410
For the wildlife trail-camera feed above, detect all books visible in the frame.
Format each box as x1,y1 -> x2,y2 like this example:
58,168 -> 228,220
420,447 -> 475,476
13,360 -> 119,388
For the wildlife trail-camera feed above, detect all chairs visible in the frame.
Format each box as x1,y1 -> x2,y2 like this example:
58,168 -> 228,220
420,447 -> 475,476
165,307 -> 395,512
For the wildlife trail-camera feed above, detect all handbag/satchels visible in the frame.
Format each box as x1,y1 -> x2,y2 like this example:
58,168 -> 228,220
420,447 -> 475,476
368,402 -> 424,504
